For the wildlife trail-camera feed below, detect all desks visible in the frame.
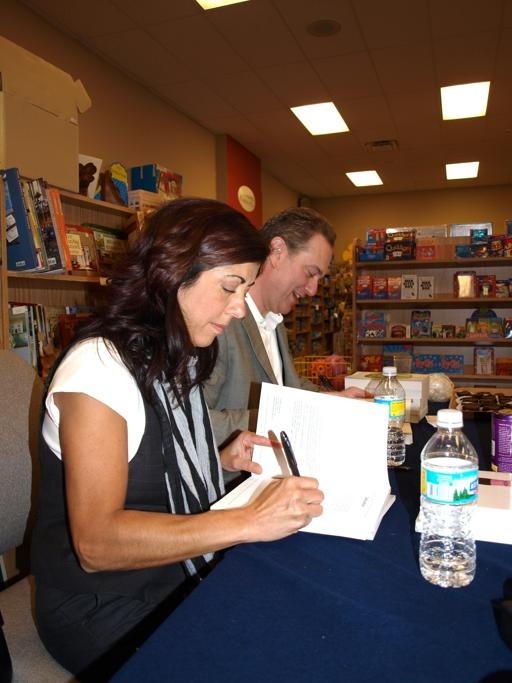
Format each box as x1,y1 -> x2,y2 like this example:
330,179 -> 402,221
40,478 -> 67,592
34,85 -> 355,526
121,389 -> 511,680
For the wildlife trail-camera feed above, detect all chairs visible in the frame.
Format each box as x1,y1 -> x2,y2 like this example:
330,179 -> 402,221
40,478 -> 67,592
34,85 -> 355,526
2,346 -> 47,626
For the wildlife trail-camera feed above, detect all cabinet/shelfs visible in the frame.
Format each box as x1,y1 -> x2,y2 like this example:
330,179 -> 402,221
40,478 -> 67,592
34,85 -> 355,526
283,274 -> 338,357
353,226 -> 512,387
0,169 -> 138,388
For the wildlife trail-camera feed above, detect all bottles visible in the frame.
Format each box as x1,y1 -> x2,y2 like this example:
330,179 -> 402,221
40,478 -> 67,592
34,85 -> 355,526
373,365 -> 406,467
417,407 -> 481,589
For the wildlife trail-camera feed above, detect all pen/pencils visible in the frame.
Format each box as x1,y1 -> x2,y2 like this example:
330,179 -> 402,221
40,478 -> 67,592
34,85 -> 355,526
319,375 -> 336,391
267,430 -> 291,477
280,431 -> 301,476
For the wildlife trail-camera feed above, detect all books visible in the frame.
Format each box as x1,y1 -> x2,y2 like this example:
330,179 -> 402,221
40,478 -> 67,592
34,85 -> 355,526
211,380 -> 391,540
7,302 -> 93,384
345,371 -> 432,425
367,494 -> 397,540
415,466 -> 510,546
2,161 -> 184,275
356,270 -> 512,375
414,237 -> 435,261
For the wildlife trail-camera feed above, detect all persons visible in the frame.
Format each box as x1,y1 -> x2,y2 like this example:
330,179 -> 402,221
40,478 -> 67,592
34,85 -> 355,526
26,196 -> 324,682
200,205 -> 380,490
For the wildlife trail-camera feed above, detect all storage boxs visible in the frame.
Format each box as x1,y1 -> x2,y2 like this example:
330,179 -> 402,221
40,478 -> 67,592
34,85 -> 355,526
1,34 -> 83,193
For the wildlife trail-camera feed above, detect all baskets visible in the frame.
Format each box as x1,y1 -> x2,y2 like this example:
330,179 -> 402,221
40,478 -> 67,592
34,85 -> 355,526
293,356 -> 352,385
